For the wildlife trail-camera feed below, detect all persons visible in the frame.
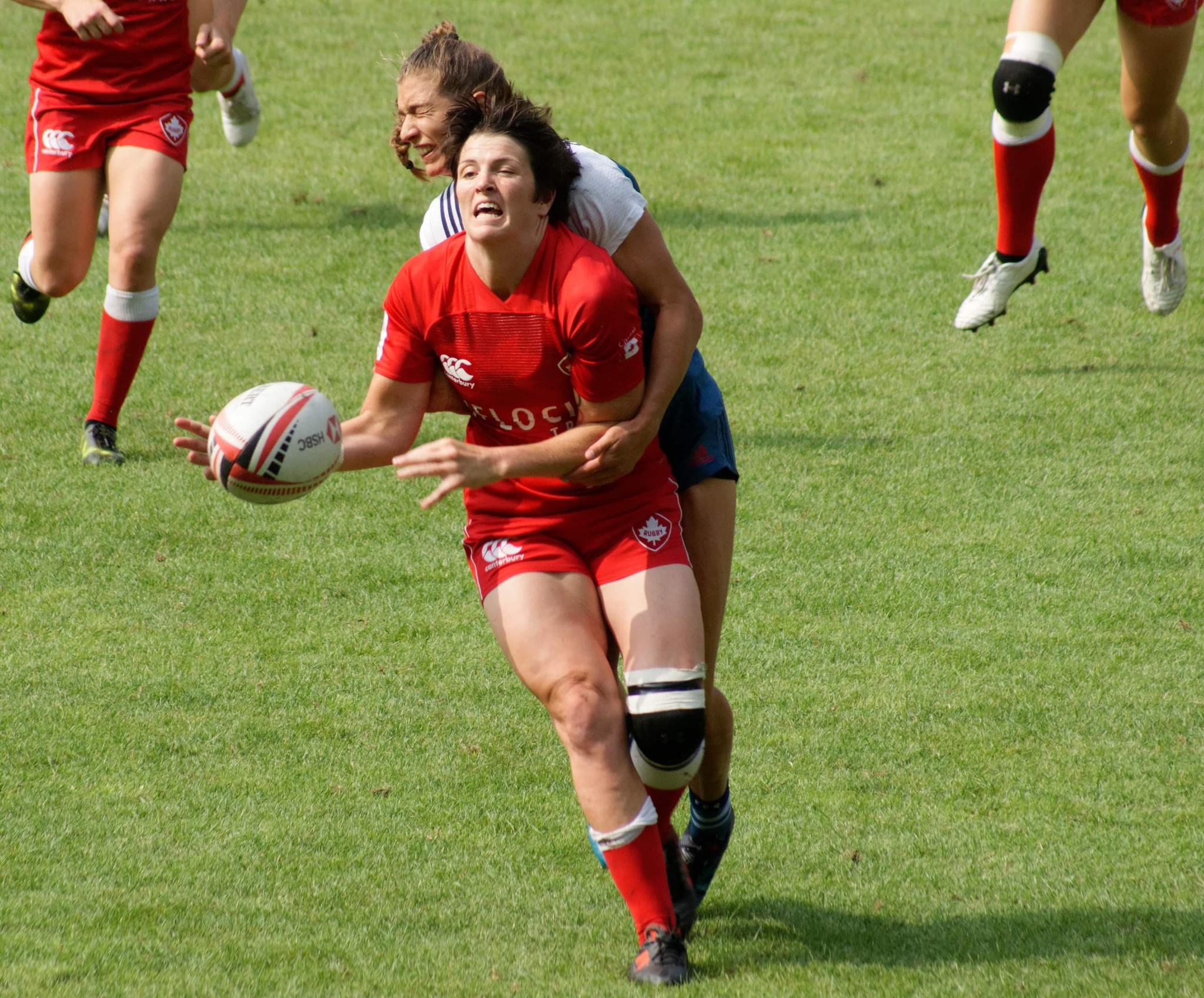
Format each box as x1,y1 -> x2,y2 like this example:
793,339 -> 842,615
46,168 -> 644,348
97,0 -> 261,235
12,0 -> 247,464
954,0 -> 1202,332
174,97 -> 705,986
387,21 -> 739,904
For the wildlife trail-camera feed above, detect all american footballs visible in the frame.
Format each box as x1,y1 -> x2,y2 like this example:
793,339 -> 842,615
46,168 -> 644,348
205,382 -> 343,507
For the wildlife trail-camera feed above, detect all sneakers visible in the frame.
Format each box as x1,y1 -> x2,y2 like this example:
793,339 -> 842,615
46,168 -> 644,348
80,420 -> 126,465
216,49 -> 263,147
953,234 -> 1051,334
662,832 -> 695,942
676,810 -> 740,907
10,230 -> 52,326
1141,203 -> 1189,317
631,912 -> 690,990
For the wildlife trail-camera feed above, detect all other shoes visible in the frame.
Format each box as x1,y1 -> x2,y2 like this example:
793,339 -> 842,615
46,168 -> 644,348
95,195 -> 111,237
